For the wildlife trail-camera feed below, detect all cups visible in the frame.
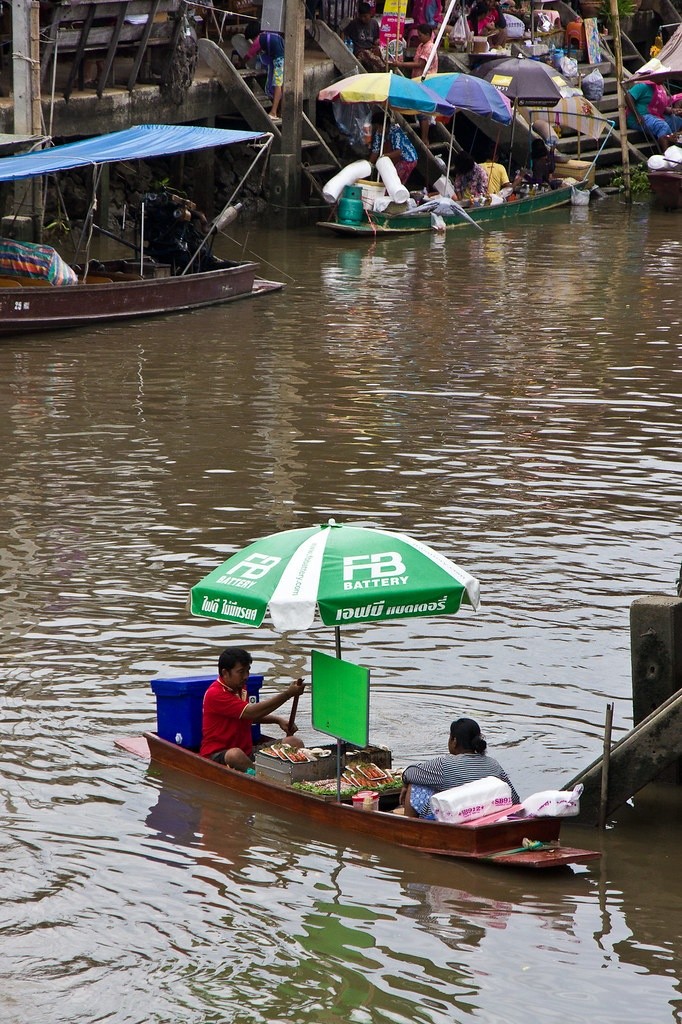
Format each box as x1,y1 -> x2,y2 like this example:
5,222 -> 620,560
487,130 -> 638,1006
474,198 -> 478,207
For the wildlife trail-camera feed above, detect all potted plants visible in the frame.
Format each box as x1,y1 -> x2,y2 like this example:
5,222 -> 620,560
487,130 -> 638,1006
600,0 -> 637,34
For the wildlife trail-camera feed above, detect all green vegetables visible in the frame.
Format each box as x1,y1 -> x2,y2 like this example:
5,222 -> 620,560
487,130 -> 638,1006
292,776 -> 404,796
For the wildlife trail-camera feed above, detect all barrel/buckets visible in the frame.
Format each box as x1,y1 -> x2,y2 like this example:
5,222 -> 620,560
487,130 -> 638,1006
352,791 -> 379,810
474,37 -> 487,53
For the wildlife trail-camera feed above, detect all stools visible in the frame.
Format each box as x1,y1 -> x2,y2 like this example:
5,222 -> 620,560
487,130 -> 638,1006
534,9 -> 561,29
407,28 -> 435,46
565,21 -> 587,49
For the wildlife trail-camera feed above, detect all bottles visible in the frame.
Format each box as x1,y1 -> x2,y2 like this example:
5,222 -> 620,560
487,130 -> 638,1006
554,50 -> 561,67
344,39 -> 350,51
470,195 -> 474,208
485,193 -> 492,205
479,193 -> 483,206
549,42 -> 556,59
532,184 -> 536,190
255,55 -> 261,70
561,48 -> 577,59
423,187 -> 428,195
450,36 -> 467,53
499,0 -> 507,4
536,183 -> 538,190
349,39 -> 353,53
485,196 -> 491,206
466,37 -> 474,53
444,33 -> 449,53
526,185 -> 530,197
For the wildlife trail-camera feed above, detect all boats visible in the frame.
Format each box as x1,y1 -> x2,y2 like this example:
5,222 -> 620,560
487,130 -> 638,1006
648,160 -> 682,207
317,108 -> 614,235
0,126 -> 290,334
112,726 -> 604,870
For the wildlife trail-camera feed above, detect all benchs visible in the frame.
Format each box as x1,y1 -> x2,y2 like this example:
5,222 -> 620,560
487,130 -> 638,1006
0,272 -> 143,286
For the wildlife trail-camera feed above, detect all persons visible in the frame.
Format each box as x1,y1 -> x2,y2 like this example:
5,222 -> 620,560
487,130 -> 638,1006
625,73 -> 682,153
235,22 -> 285,118
199,647 -> 305,772
393,0 -> 516,79
340,2 -> 382,60
433,137 -> 556,201
369,111 -> 418,196
399,718 -> 521,821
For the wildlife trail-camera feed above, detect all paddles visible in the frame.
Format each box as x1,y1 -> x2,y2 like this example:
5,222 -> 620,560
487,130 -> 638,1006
284,678 -> 306,741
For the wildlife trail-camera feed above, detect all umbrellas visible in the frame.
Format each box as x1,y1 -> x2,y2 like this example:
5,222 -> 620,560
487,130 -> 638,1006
185,518 -> 481,766
319,54 -> 607,197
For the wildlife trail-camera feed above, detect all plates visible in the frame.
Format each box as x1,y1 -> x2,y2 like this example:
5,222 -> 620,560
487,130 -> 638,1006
258,742 -> 402,788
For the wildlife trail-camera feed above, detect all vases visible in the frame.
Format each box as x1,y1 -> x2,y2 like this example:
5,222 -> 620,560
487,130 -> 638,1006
632,0 -> 642,12
581,0 -> 605,17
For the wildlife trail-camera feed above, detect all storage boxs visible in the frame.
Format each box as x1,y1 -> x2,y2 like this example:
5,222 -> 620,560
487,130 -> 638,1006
151,674 -> 264,749
124,262 -> 171,279
354,180 -> 386,210
553,160 -> 596,189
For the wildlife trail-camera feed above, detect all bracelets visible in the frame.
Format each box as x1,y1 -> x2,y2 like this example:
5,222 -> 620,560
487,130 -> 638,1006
283,690 -> 290,701
